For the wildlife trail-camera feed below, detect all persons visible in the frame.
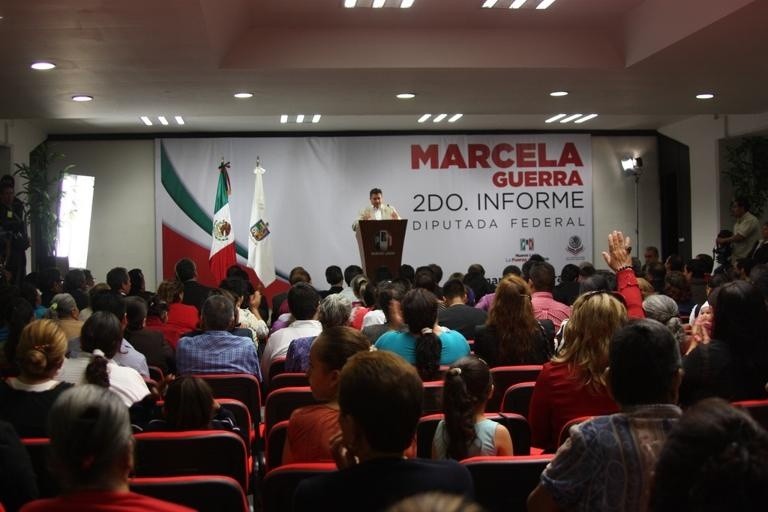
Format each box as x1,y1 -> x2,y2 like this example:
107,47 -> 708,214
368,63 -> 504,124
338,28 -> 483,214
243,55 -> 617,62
361,288 -> 411,348
128,268 -> 155,300
0,297 -> 35,371
175,258 -> 215,318
339,264 -> 363,303
553,263 -> 581,307
261,283 -> 323,382
271,266 -> 305,326
526,229 -> 645,453
0,317 -> 76,438
106,266 -> 132,299
439,279 -> 488,340
54,312 -> 152,407
0,184 -> 32,290
77,282 -> 112,321
361,281 -> 391,330
281,325 -> 418,464
293,349 -> 483,512
667,258 -> 709,314
147,280 -> 201,348
125,295 -> 174,372
278,271 -> 312,317
348,282 -> 378,330
464,264 -> 497,303
642,245 -> 667,294
473,273 -> 552,369
686,301 -> 714,356
734,255 -> 754,285
0,175 -> 24,220
317,264 -> 344,300
678,278 -> 768,407
578,260 -> 609,295
746,219 -> 767,264
716,195 -> 760,266
285,292 -> 352,373
18,383 -> 198,512
374,288 -> 471,367
220,278 -> 270,352
474,265 -> 522,311
163,374 -> 238,428
352,275 -> 370,308
68,289 -> 151,379
174,295 -> 261,376
526,261 -> 572,331
648,396 -> 768,512
527,316 -> 685,512
351,188 -> 403,232
46,292 -> 85,339
430,353 -> 514,462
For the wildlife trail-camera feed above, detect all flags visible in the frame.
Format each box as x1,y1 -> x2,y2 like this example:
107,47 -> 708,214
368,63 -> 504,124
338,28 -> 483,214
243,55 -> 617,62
247,157 -> 276,288
209,155 -> 237,287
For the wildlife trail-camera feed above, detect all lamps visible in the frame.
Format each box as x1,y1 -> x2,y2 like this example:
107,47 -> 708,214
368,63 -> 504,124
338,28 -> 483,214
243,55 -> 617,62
481,0 -> 498,9
545,112 -> 566,124
174,115 -> 184,125
311,114 -> 321,123
417,114 -> 432,123
400,0 -> 414,9
29,62 -> 55,70
344,0 -> 357,9
396,93 -> 416,100
433,113 -> 447,124
157,115 -> 168,126
574,113 -> 599,123
535,0 -> 557,10
549,91 -> 568,97
371,0 -> 387,8
73,95 -> 92,102
139,115 -> 153,126
620,157 -> 644,174
560,114 -> 583,124
234,92 -> 253,98
509,0 -> 527,9
448,113 -> 463,123
296,114 -> 304,124
280,114 -> 288,124
696,94 -> 714,100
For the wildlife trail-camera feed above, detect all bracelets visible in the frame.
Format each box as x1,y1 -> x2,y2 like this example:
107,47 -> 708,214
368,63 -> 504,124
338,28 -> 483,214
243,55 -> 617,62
615,265 -> 636,273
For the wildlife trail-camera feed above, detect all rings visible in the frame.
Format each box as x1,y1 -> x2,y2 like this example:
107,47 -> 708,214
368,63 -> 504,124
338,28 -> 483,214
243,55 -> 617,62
625,247 -> 632,254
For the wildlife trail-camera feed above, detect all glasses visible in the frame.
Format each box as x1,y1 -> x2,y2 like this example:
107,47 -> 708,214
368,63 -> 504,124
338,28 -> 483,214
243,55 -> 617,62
580,289 -> 627,312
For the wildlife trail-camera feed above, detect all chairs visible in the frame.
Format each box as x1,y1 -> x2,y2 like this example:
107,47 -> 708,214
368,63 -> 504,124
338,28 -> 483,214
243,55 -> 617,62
152,397 -> 256,457
184,371 -> 263,435
266,384 -> 334,423
255,464 -> 354,511
501,382 -> 540,414
128,474 -> 250,511
128,430 -> 253,492
454,454 -> 555,512
14,437 -> 115,487
268,367 -> 315,386
489,363 -> 547,410
418,378 -> 478,411
414,413 -> 533,456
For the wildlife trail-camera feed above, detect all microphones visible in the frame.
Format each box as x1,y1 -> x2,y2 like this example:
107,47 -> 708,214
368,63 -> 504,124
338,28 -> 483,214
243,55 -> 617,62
387,204 -> 398,219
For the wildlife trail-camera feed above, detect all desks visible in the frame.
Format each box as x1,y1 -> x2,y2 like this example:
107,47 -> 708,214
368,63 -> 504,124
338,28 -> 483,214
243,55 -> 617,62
354,219 -> 407,287
